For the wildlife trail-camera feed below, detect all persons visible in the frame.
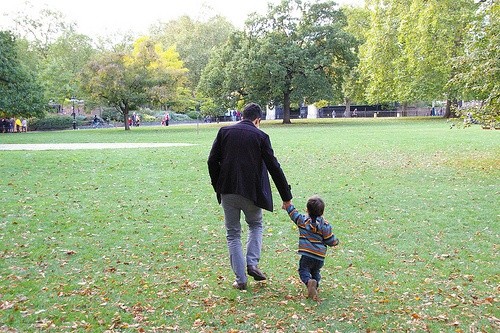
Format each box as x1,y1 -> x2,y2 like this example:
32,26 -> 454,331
0,116 -> 28,132
160,113 -> 171,125
332,109 -> 336,117
206,102 -> 293,291
435,105 -> 448,115
231,106 -> 245,120
282,196 -> 340,300
352,108 -> 359,118
129,111 -> 140,126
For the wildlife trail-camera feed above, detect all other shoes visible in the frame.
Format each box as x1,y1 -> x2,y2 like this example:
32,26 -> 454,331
232,281 -> 247,288
307,279 -> 317,298
247,267 -> 266,280
